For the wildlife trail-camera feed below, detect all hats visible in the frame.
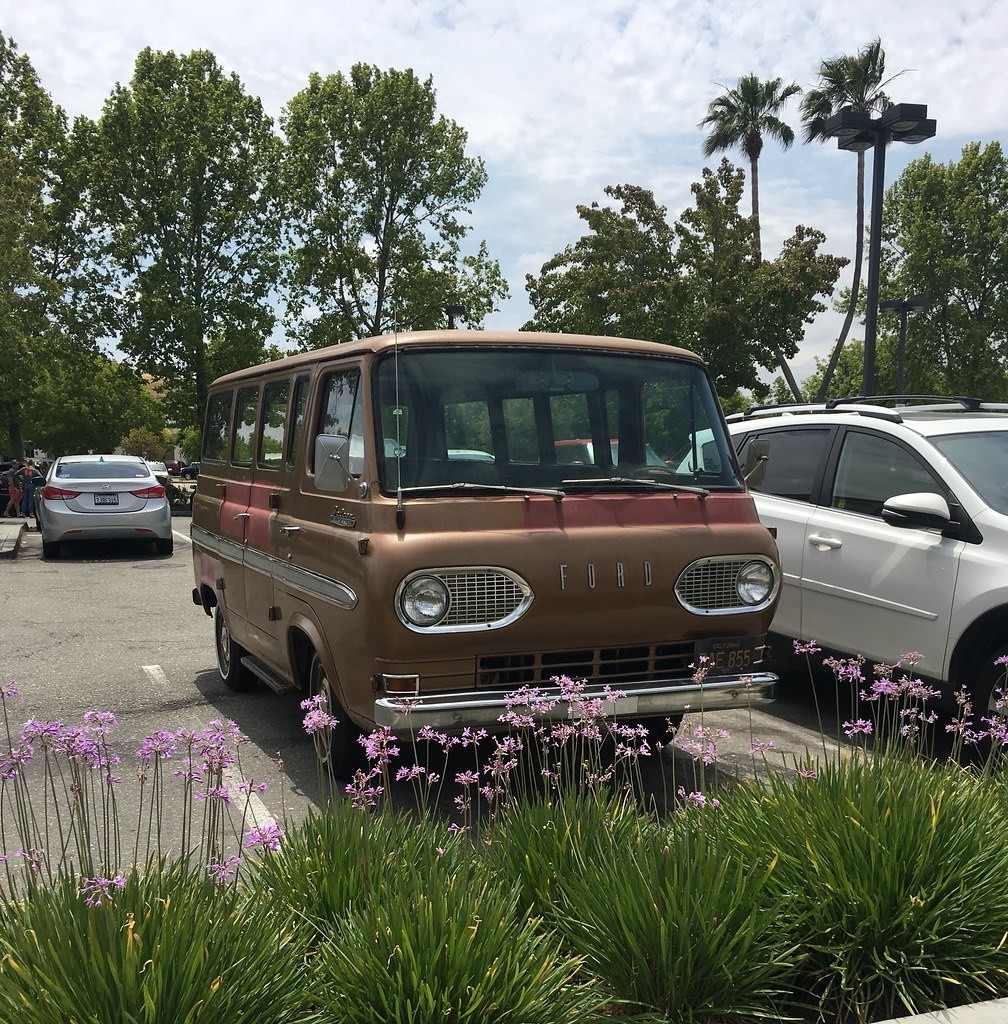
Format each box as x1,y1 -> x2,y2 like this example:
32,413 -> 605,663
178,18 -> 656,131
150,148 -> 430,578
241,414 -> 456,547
11,459 -> 20,464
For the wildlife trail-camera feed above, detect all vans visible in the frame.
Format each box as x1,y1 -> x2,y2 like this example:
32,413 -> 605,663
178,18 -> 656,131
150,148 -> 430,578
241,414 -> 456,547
189,325 -> 783,782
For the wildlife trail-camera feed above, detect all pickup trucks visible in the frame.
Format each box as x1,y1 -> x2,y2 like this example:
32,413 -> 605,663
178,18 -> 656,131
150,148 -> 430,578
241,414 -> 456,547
180,466 -> 200,480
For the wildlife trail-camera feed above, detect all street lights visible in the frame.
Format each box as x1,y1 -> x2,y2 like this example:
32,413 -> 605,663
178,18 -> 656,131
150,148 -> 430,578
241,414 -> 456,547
879,299 -> 929,405
826,102 -> 937,397
446,305 -> 467,448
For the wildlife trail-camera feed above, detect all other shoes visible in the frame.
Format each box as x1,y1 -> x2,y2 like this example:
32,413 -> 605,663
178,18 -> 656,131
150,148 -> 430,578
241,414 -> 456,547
29,513 -> 35,518
2,511 -> 12,518
17,513 -> 26,518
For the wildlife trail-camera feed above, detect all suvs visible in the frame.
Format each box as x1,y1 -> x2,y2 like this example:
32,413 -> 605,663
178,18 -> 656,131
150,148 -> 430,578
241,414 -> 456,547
678,395 -> 1008,774
552,440 -> 676,473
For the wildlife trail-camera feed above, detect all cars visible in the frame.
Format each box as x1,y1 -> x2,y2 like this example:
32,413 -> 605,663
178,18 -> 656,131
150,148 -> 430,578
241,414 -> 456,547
446,449 -> 496,460
31,453 -> 173,558
145,461 -> 172,484
164,460 -> 185,477
0,461 -> 45,517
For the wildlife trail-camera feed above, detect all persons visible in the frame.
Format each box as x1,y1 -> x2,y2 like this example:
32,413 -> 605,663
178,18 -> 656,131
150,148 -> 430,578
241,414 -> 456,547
3,459 -> 49,518
